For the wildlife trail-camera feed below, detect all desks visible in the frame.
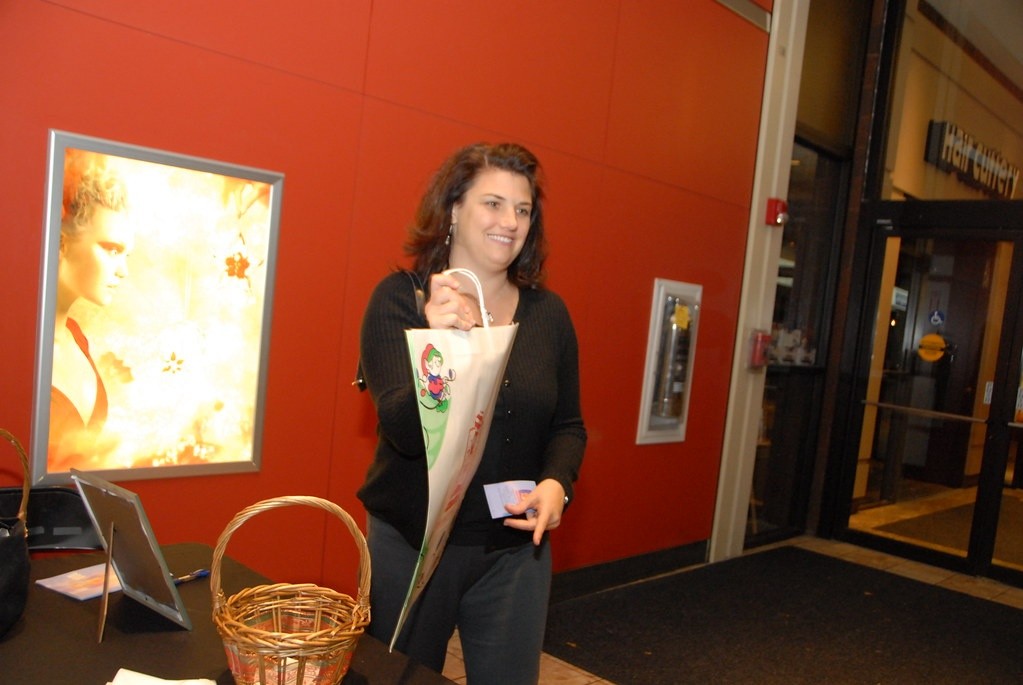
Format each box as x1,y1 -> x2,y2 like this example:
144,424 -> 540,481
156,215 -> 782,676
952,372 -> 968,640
0,542 -> 463,685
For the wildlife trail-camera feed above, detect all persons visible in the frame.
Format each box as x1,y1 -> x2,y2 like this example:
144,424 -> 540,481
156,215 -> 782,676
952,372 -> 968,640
359,143 -> 587,685
47,149 -> 269,473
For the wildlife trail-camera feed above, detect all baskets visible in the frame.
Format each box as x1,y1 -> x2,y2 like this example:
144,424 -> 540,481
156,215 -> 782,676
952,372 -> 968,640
210,496 -> 371,685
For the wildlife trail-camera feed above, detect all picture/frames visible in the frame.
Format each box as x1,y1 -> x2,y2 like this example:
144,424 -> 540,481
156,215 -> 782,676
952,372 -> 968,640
28,128 -> 285,489
69,467 -> 193,643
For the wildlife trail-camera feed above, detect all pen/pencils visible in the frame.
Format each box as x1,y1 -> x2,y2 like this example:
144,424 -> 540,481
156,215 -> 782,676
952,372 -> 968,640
173,568 -> 212,587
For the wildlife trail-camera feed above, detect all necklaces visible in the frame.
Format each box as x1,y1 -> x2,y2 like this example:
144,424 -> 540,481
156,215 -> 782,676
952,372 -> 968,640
488,312 -> 494,323
